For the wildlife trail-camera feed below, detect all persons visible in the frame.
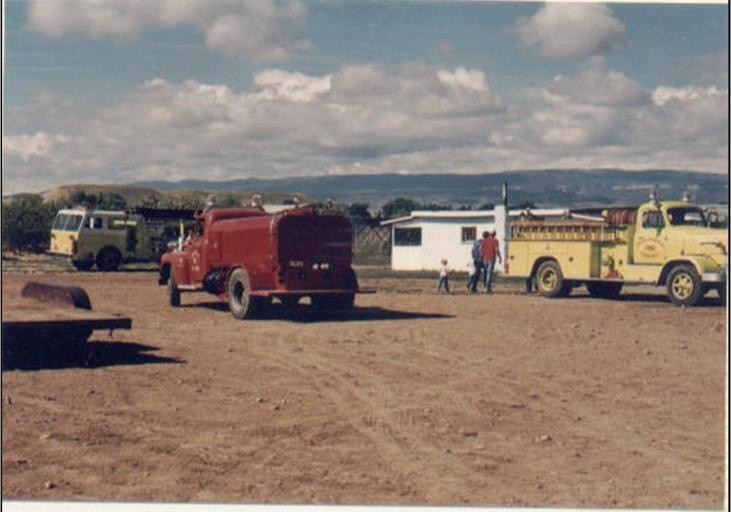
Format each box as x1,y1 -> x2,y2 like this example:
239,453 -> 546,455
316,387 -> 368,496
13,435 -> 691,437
465,231 -> 490,292
434,258 -> 455,293
160,226 -> 194,254
479,229 -> 503,294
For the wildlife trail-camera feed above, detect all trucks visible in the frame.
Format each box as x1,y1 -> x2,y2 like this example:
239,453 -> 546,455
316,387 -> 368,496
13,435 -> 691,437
45,210 -> 168,270
507,201 -> 728,307
159,208 -> 358,319
